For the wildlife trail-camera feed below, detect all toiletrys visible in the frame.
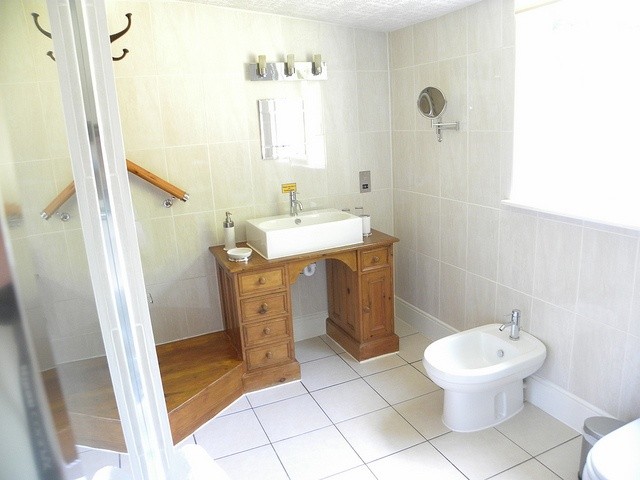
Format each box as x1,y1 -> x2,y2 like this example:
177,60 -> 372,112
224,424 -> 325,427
224,211 -> 237,250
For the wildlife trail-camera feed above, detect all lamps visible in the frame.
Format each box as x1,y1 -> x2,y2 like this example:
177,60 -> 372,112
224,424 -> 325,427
287,54 -> 296,77
257,55 -> 268,76
314,54 -> 322,75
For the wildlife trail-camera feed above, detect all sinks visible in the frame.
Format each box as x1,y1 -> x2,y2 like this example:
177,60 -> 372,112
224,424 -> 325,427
423,323 -> 547,391
245,208 -> 364,260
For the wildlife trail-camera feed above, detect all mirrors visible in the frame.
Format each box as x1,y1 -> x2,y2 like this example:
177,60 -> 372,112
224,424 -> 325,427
418,86 -> 447,119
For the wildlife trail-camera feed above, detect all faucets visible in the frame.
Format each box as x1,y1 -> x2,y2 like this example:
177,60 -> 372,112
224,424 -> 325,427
499,322 -> 514,331
290,190 -> 303,216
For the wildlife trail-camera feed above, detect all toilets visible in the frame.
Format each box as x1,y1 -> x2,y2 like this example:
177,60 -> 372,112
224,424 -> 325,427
582,417 -> 640,479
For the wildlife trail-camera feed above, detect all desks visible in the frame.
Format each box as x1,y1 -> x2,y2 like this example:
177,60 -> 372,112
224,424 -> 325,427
209,228 -> 398,371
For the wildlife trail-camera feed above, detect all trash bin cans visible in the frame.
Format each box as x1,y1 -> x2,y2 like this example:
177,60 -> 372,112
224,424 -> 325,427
578,416 -> 629,480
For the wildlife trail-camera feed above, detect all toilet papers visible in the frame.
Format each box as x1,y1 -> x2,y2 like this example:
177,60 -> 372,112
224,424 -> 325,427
363,216 -> 372,234
